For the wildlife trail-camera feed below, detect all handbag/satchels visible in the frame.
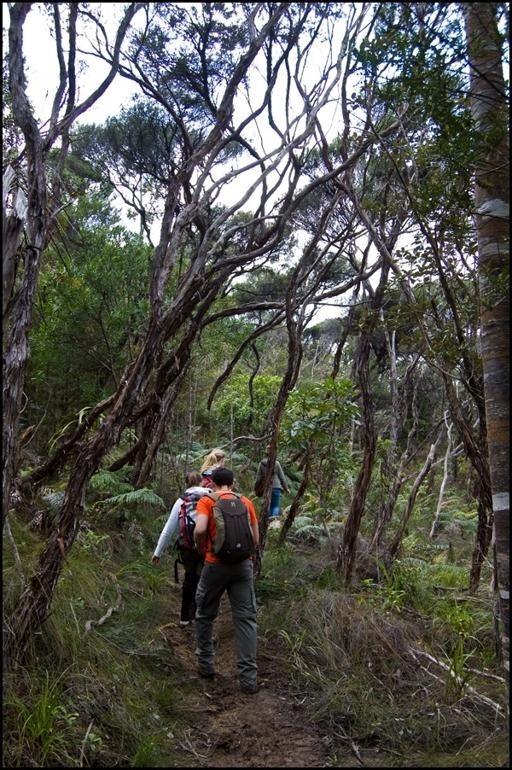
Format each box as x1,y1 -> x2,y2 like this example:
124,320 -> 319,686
255,481 -> 264,497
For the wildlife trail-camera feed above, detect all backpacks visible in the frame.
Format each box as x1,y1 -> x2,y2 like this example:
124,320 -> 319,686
179,490 -> 211,549
204,493 -> 256,562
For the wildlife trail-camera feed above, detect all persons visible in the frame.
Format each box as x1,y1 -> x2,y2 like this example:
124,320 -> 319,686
150,470 -> 214,654
198,448 -> 226,489
257,445 -> 291,516
193,466 -> 260,693
